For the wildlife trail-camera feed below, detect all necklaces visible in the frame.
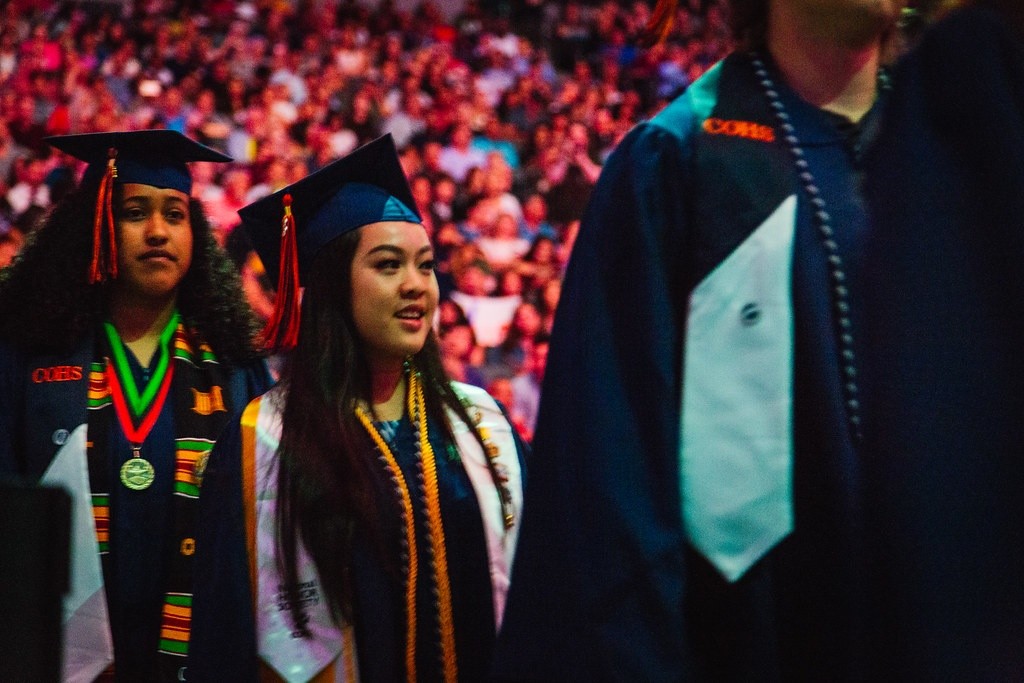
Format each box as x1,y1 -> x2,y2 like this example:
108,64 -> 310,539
748,55 -> 896,465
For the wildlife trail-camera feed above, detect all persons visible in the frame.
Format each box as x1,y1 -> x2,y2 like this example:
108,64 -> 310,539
179,133 -> 535,683
488,2 -> 909,681
877,1 -> 1024,683
0,130 -> 279,683
0,1 -> 736,443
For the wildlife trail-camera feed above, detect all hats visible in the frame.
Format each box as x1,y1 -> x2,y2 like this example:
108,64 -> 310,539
236,132 -> 423,353
42,130 -> 235,284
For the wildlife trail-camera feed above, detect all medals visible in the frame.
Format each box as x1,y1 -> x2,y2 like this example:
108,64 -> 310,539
120,456 -> 155,492
